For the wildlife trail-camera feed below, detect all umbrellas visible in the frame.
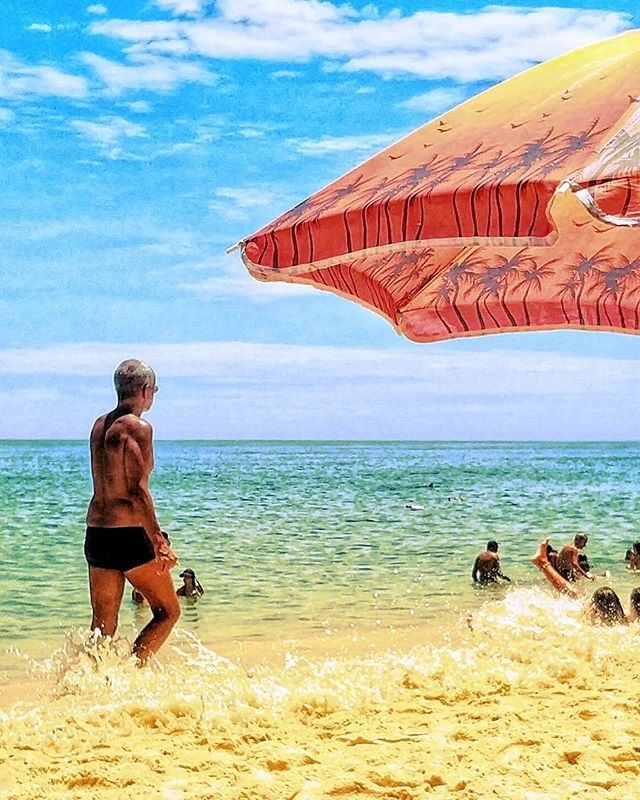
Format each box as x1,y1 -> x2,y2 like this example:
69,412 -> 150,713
221,30 -> 640,345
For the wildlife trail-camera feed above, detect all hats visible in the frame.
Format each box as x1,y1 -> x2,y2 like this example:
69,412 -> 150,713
179,569 -> 195,578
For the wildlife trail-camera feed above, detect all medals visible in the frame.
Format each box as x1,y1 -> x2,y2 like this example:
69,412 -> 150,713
545,544 -> 640,582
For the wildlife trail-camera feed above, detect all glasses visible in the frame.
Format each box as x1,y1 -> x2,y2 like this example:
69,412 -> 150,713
148,385 -> 159,393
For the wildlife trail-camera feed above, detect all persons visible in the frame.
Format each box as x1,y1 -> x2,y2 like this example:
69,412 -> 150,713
472,540 -> 511,584
529,535 -> 640,623
554,533 -> 596,584
176,569 -> 202,599
583,586 -> 629,627
160,531 -> 171,547
85,357 -> 180,667
